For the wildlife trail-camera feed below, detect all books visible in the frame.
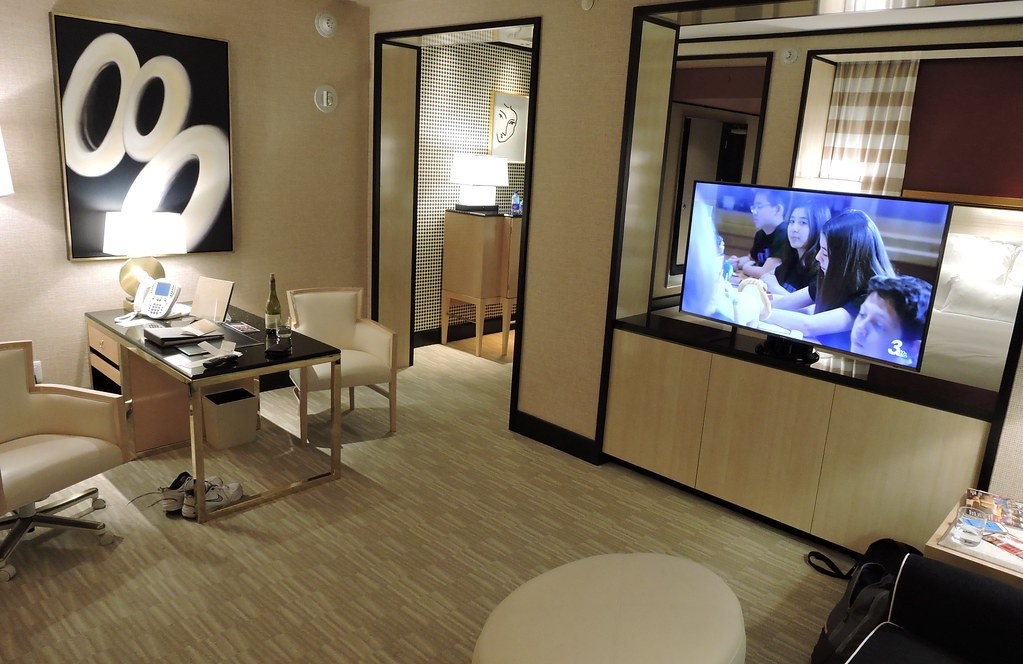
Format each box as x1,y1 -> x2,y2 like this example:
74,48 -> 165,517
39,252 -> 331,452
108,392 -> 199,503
144,319 -> 225,348
982,532 -> 1023,559
966,487 -> 1023,529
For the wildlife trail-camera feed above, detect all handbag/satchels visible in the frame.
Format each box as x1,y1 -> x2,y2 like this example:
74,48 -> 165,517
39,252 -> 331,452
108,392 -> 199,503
807,538 -> 924,580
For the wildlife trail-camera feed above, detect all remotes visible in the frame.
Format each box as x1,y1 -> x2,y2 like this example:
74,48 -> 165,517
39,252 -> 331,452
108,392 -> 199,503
202,354 -> 240,370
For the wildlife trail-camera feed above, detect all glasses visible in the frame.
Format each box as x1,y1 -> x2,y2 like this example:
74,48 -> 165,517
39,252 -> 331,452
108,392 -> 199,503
750,203 -> 772,212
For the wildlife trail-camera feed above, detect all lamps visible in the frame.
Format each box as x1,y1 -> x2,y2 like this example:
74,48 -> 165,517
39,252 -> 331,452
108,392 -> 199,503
452,152 -> 509,211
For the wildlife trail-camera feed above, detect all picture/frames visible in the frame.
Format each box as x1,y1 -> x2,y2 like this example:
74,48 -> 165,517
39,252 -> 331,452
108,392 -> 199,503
48,10 -> 236,260
488,91 -> 530,163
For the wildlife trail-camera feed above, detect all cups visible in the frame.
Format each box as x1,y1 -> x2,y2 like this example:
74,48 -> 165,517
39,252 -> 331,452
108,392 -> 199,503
953,506 -> 987,547
276,316 -> 292,338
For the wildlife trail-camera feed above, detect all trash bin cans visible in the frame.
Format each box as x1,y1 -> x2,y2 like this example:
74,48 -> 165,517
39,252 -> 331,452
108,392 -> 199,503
202,387 -> 257,450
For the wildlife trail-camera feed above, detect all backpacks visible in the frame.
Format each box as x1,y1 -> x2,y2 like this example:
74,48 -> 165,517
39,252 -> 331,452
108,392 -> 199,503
810,562 -> 896,664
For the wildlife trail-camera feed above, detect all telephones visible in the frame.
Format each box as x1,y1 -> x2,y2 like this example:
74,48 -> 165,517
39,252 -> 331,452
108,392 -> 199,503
134,278 -> 182,321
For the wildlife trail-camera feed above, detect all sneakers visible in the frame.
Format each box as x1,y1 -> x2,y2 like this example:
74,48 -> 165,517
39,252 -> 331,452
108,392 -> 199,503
181,482 -> 243,519
127,471 -> 223,512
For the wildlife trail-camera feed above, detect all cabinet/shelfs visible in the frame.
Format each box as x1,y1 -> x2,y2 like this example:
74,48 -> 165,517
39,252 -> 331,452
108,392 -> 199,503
595,314 -> 1006,558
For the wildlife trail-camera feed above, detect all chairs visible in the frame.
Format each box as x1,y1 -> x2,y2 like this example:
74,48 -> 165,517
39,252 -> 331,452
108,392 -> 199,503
285,286 -> 398,448
0,342 -> 138,582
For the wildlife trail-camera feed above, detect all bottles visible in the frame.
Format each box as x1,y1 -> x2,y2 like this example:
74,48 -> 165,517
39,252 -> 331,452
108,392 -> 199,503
512,191 -> 519,215
264,273 -> 282,333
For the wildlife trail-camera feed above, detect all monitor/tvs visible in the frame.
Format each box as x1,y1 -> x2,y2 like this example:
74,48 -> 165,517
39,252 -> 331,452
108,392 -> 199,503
678,180 -> 954,374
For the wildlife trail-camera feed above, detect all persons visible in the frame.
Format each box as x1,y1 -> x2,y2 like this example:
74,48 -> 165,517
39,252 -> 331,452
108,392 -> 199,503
850,275 -> 933,368
496,103 -> 518,143
717,191 -> 896,352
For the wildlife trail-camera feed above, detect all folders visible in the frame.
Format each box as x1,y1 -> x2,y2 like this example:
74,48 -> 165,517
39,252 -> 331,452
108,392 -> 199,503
143,326 -> 225,348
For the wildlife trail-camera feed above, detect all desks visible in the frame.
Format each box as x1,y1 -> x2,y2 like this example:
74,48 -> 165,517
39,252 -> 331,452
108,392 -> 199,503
84,301 -> 343,525
923,493 -> 1023,591
442,210 -> 524,359
471,553 -> 746,664
